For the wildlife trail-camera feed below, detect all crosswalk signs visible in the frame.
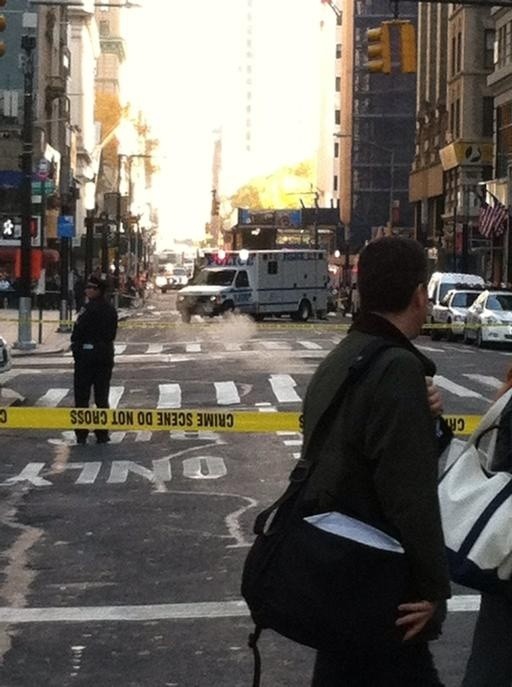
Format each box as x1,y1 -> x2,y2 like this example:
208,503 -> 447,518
0,211 -> 24,247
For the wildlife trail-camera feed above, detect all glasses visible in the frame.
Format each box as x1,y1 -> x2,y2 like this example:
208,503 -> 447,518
87,285 -> 97,289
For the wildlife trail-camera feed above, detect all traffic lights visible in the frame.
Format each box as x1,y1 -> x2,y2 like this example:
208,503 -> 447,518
334,227 -> 345,258
30,215 -> 43,248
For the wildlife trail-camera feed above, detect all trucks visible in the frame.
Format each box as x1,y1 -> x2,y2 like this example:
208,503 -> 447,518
153,249 -> 329,322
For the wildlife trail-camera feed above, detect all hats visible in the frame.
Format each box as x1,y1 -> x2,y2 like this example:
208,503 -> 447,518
87,275 -> 108,290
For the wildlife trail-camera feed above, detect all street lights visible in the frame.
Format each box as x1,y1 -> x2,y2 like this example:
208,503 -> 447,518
114,154 -> 159,287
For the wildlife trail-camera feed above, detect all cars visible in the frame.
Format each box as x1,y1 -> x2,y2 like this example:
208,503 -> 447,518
420,272 -> 512,347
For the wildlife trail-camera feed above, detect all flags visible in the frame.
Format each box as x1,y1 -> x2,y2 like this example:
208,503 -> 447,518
493,196 -> 509,236
478,201 -> 494,239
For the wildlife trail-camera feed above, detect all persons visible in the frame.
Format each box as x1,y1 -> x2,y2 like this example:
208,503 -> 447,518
333,281 -> 361,316
459,370 -> 511,687
301,237 -> 451,686
72,276 -> 118,444
0,267 -> 146,312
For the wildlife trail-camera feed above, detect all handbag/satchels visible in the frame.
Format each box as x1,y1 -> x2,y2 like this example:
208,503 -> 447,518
437,437 -> 512,598
241,491 -> 449,656
